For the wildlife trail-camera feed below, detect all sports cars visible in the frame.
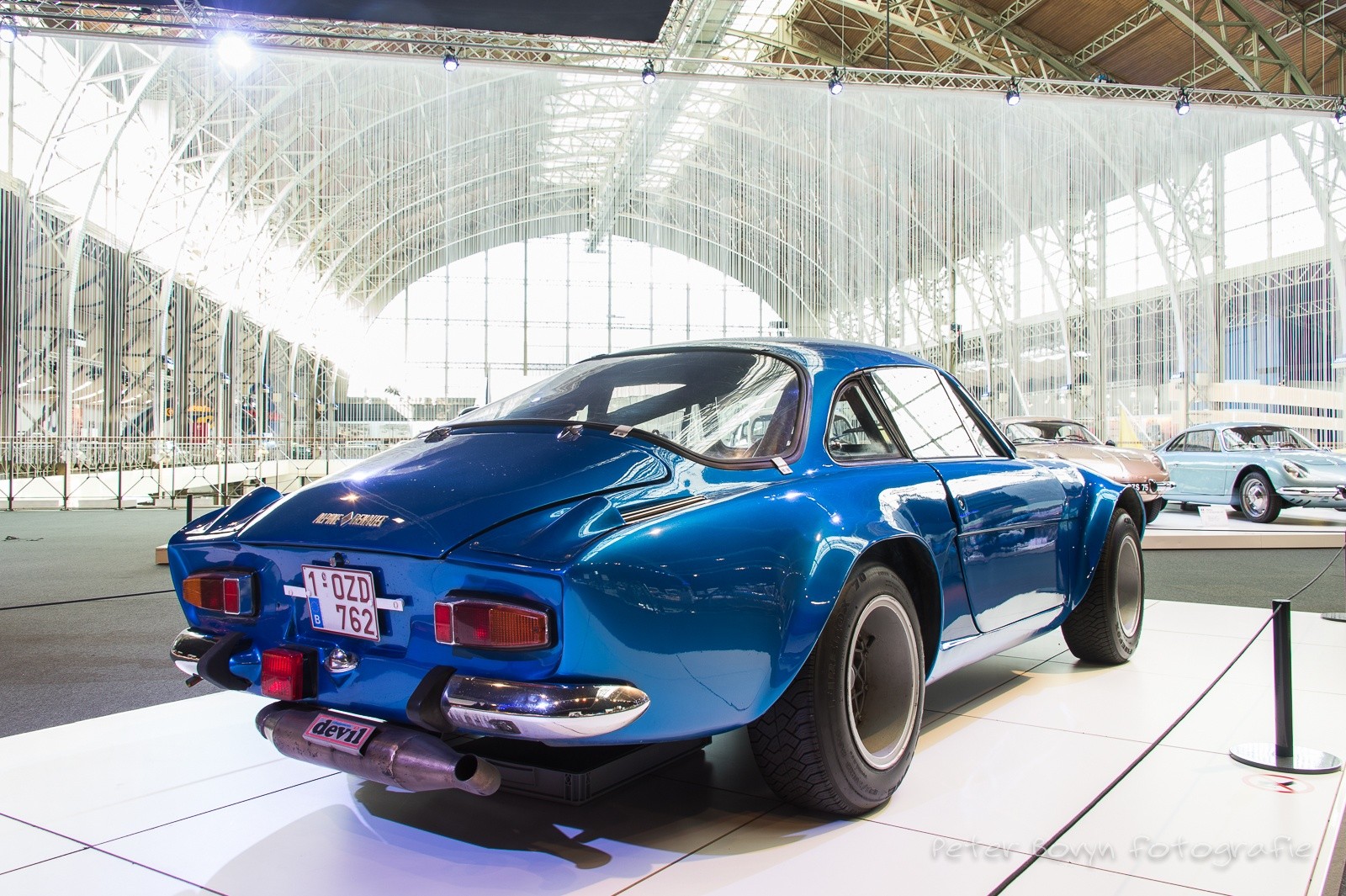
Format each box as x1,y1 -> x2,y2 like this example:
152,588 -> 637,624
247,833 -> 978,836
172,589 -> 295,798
1152,422 -> 1346,522
995,415 -> 1175,523
168,338 -> 1149,816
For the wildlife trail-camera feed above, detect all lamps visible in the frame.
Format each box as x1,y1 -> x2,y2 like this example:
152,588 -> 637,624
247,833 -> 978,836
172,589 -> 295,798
443,45 -> 461,71
641,60 -> 657,83
0,15 -> 18,42
1334,94 -> 1346,124
828,66 -> 843,94
1005,75 -> 1021,104
1175,84 -> 1190,115
